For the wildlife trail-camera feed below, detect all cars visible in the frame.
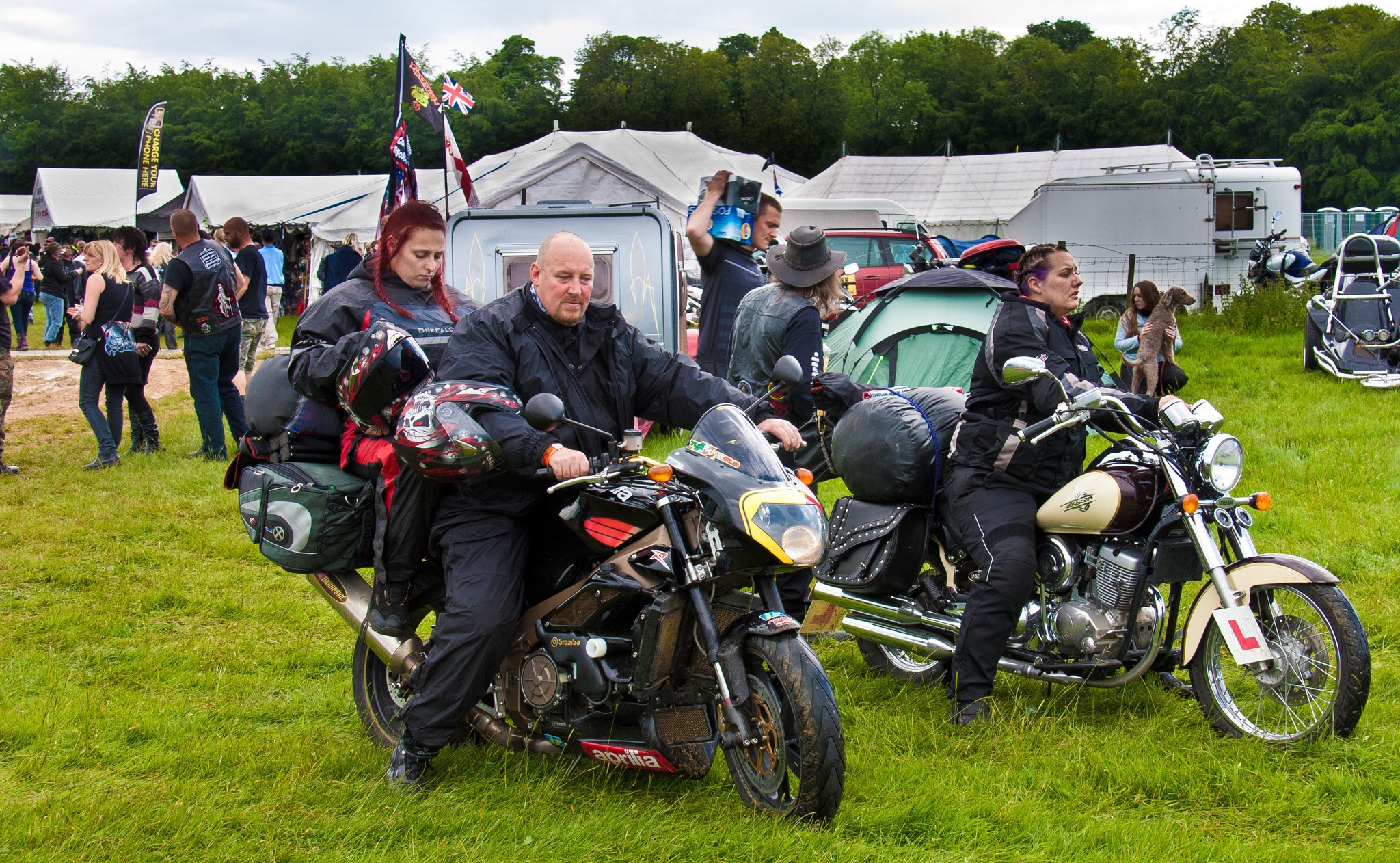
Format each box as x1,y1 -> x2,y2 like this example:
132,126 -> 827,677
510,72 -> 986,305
782,228 -> 946,324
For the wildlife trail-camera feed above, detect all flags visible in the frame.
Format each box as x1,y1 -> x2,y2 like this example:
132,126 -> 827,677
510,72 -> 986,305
439,73 -> 475,118
136,99 -> 166,198
395,32 -> 445,137
372,114 -> 419,234
442,109 -> 481,208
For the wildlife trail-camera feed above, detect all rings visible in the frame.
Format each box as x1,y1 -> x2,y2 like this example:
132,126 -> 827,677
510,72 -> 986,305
141,349 -> 144,352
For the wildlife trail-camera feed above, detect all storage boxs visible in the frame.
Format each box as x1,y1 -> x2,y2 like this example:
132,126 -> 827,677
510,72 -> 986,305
685,175 -> 762,246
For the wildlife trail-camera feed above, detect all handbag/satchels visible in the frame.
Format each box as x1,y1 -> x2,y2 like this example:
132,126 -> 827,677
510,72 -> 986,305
68,337 -> 99,366
812,494 -> 933,595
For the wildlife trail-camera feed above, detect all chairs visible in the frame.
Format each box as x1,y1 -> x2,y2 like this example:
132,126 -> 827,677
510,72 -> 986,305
1336,283 -> 1388,343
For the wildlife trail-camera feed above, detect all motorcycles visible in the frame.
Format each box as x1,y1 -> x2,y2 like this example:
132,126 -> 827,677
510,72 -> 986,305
1304,233 -> 1400,390
808,357 -> 1371,753
1236,211 -> 1343,300
304,355 -> 846,830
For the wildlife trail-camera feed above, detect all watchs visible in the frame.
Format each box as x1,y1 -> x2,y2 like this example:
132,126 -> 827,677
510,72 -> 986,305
542,443 -> 563,467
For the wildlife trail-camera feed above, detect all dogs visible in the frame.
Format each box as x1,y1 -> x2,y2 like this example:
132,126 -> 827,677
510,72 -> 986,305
1119,286 -> 1196,397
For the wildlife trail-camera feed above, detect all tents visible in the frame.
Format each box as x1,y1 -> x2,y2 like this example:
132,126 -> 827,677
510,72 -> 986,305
306,111 -> 811,316
819,263 -> 1126,400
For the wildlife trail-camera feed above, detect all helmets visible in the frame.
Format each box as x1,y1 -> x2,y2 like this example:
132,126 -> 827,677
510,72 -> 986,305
392,380 -> 525,486
335,320 -> 436,436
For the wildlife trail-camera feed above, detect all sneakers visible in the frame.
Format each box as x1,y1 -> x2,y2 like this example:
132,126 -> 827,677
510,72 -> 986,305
949,700 -> 989,725
365,573 -> 413,638
1142,671 -> 1193,697
81,454 -> 121,469
386,742 -> 432,801
0,465 -> 20,475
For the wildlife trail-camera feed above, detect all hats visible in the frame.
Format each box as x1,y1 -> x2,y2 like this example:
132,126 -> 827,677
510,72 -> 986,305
766,225 -> 848,287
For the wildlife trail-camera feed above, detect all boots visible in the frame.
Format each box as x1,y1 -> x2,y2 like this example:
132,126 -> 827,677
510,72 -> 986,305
142,409 -> 161,456
121,410 -> 146,456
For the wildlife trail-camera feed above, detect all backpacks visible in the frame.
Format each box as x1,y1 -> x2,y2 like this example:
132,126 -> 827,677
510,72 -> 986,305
238,462 -> 375,574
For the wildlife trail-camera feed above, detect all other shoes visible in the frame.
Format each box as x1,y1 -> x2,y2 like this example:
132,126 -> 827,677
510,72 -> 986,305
1,303 -> 286,354
800,630 -> 852,644
187,446 -> 204,457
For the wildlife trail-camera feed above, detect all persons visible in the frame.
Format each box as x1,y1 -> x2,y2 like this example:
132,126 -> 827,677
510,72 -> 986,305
386,232 -> 805,791
2,218 -> 362,395
725,224 -> 848,640
1114,280 -> 1190,398
222,217 -> 271,410
109,223 -> 166,458
66,238 -> 135,472
686,170 -> 783,381
286,200 -> 491,635
158,208 -> 253,464
944,246 -> 1206,738
0,234 -> 30,476
